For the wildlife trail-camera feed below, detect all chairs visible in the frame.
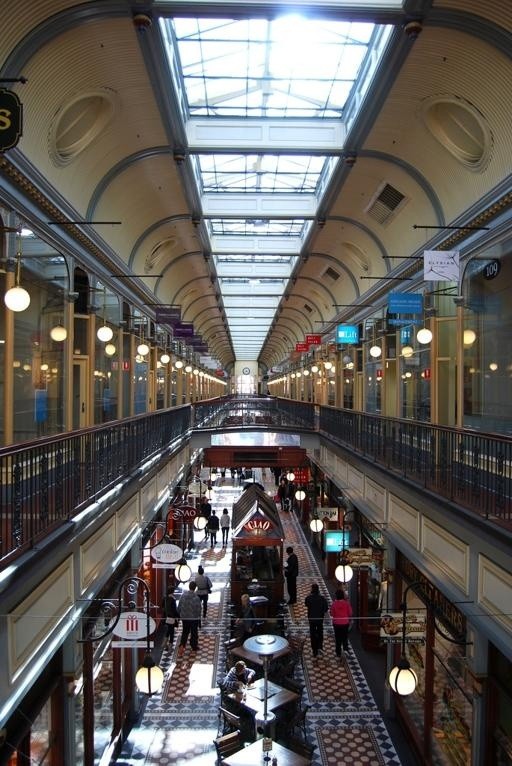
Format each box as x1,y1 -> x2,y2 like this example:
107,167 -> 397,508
212,634 -> 317,766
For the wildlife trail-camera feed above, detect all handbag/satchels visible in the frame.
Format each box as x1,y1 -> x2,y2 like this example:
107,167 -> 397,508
206,577 -> 211,593
166,616 -> 175,624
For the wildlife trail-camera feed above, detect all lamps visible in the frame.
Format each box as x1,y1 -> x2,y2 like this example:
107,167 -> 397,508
4,231 -> 30,312
169,478 -> 206,531
76,577 -> 164,696
335,509 -> 387,585
139,509 -> 191,582
309,480 -> 346,533
389,581 -> 474,696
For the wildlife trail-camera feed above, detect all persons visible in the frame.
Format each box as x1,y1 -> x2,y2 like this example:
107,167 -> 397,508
177,581 -> 201,650
220,509 -> 230,548
305,584 -> 328,656
207,510 -> 219,547
223,661 -> 256,694
330,589 -> 353,657
201,497 -> 211,538
235,594 -> 257,645
285,547 -> 298,604
277,480 -> 288,510
164,586 -> 177,650
195,565 -> 213,617
